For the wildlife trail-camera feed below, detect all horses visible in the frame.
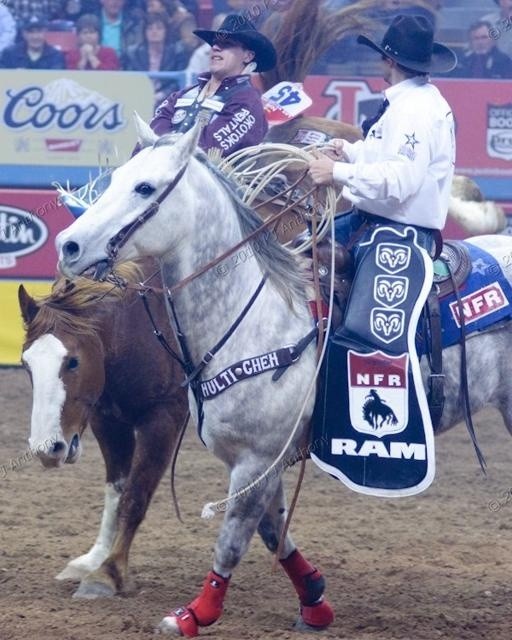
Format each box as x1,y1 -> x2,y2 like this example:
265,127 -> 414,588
52,110 -> 512,638
17,0 -> 508,602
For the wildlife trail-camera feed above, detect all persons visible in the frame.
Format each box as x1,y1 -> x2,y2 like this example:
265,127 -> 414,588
126,12 -> 274,170
303,11 -> 460,266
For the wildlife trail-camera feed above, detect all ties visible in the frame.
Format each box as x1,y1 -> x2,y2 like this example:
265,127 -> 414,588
361,98 -> 388,134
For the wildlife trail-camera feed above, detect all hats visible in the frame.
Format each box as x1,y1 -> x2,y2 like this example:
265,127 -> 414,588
356,13 -> 456,75
190,13 -> 278,71
21,12 -> 47,29
